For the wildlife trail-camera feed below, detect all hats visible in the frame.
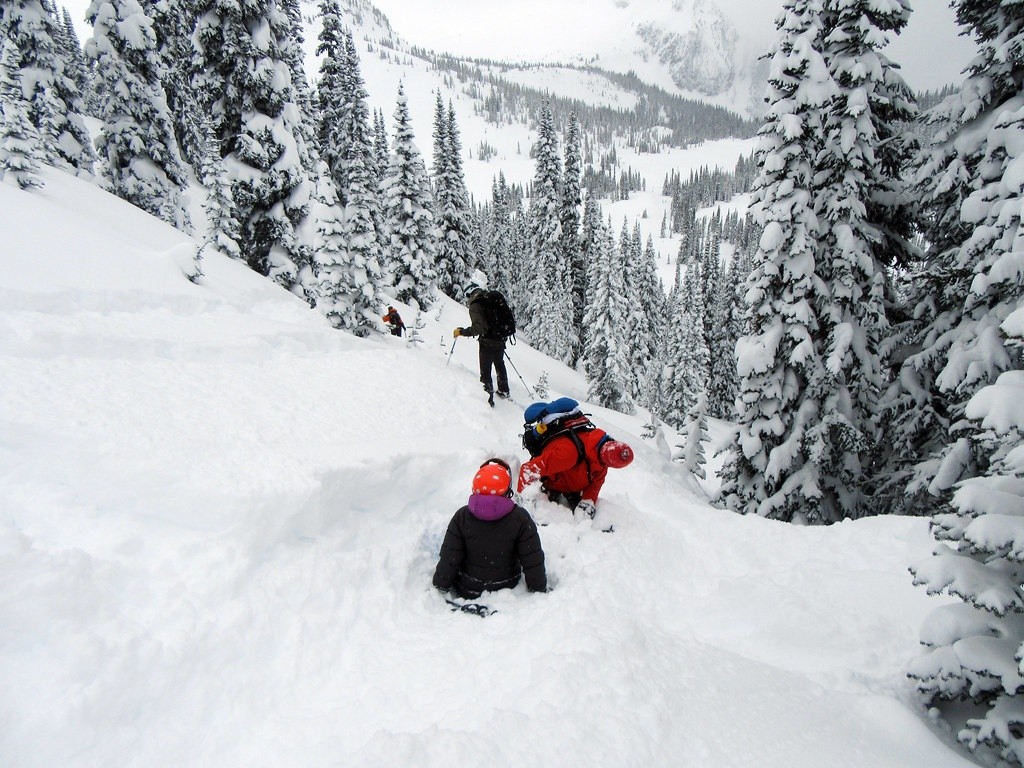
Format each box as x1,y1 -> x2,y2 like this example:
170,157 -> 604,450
473,462 -> 511,496
463,282 -> 481,296
600,441 -> 634,469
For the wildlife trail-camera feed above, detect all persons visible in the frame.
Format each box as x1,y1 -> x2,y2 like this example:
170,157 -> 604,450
430,457 -> 548,600
453,281 -> 510,407
516,413 -> 633,517
382,306 -> 407,337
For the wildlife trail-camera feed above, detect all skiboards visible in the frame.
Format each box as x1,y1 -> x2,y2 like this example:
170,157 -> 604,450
483,387 -> 529,413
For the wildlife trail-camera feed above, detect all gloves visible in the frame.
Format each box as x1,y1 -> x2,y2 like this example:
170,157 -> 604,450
454,327 -> 463,338
575,501 -> 595,520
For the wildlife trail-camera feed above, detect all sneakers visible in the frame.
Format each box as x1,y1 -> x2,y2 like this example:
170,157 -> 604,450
496,390 -> 510,398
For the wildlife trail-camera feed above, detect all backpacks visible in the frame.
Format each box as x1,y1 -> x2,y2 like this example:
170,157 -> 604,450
471,291 -> 516,339
523,397 -> 596,464
